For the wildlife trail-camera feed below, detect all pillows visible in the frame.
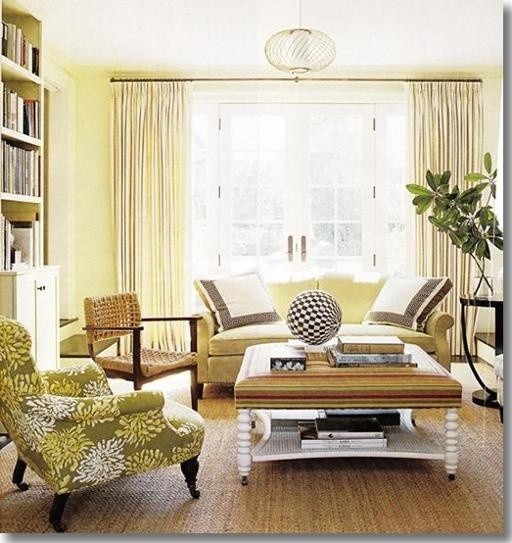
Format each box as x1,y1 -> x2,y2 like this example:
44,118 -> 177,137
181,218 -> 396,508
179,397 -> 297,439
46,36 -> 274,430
192,269 -> 285,334
360,271 -> 454,331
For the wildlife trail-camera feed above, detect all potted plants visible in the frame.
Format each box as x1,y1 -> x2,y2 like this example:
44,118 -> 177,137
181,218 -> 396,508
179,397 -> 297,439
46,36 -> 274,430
404,146 -> 504,298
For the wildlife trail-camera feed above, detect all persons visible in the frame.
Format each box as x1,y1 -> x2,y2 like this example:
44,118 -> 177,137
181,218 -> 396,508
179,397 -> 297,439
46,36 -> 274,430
272,359 -> 304,370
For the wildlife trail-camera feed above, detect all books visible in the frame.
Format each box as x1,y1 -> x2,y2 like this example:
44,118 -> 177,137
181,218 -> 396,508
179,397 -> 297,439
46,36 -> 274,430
297,409 -> 399,449
327,334 -> 417,368
2,20 -> 47,271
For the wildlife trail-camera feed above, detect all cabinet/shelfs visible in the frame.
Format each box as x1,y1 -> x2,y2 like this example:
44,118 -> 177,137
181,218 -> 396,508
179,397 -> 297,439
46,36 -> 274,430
0,265 -> 62,371
0,1 -> 50,271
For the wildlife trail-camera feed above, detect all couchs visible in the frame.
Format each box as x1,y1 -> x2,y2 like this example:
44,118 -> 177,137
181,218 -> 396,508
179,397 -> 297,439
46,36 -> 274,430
193,272 -> 456,401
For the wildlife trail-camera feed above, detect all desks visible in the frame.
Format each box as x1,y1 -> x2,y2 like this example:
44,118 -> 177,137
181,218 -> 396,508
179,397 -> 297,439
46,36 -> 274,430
234,339 -> 464,486
456,292 -> 504,408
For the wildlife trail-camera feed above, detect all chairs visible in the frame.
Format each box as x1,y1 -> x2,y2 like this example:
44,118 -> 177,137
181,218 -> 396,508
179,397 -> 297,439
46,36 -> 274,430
82,288 -> 203,413
1,312 -> 207,532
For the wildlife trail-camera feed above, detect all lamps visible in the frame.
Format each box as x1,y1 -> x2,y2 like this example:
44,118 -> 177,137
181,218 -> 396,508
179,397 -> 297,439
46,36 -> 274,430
263,1 -> 337,76
